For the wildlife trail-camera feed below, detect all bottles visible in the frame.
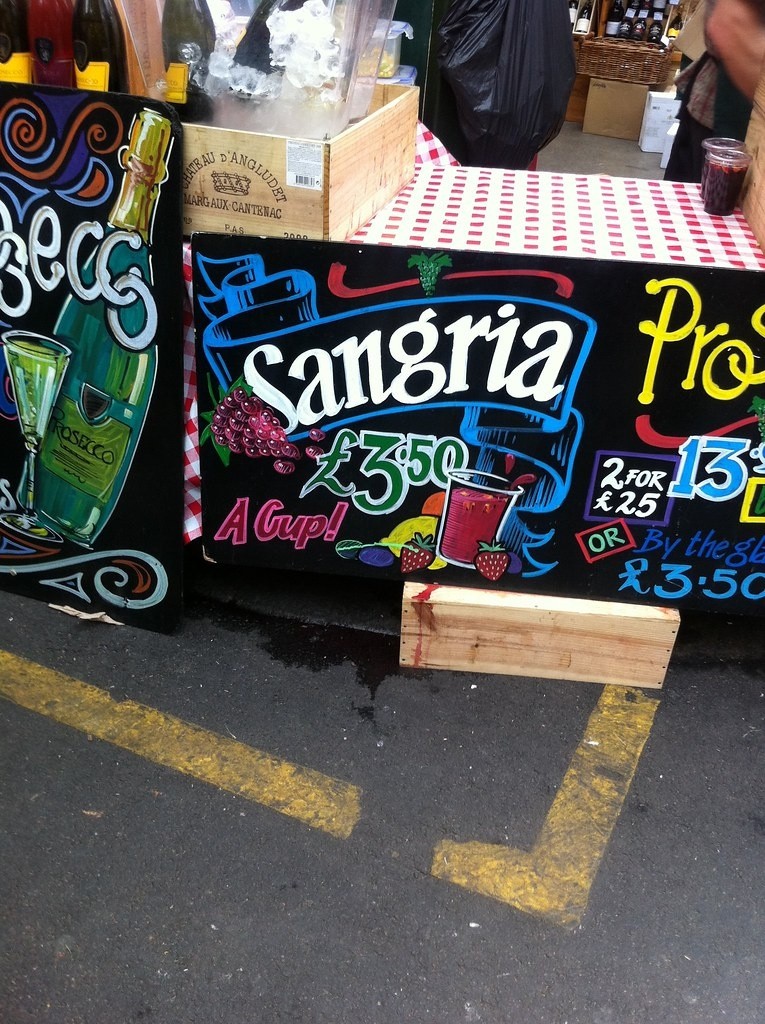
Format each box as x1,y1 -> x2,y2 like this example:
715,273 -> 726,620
569,0 -> 687,54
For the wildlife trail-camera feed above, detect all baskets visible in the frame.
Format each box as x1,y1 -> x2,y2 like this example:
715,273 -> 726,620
577,32 -> 675,85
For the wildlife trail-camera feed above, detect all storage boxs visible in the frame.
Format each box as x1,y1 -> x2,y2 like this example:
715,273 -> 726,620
358,64 -> 418,85
660,123 -> 679,169
582,79 -> 649,142
181,80 -> 419,241
637,92 -> 681,151
358,19 -> 414,78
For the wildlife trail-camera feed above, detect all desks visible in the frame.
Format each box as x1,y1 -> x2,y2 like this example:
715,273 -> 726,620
183,164 -> 765,600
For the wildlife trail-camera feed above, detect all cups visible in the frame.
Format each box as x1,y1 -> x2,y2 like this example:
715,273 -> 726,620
703,149 -> 753,216
702,137 -> 747,199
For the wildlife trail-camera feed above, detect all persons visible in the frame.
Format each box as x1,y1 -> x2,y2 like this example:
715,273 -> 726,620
663,0 -> 765,183
